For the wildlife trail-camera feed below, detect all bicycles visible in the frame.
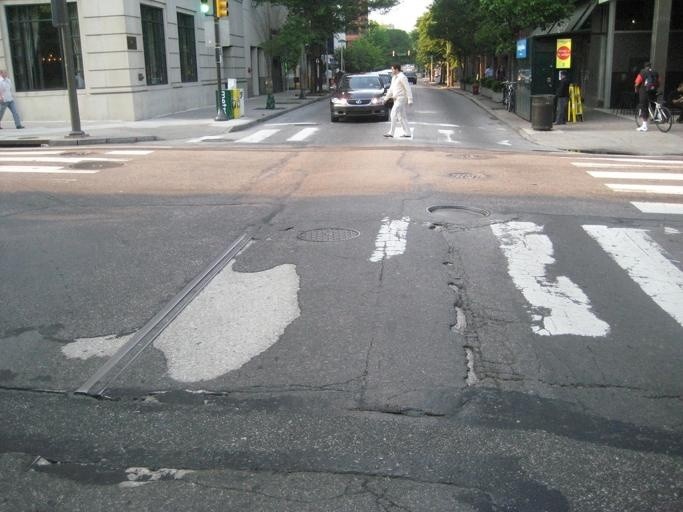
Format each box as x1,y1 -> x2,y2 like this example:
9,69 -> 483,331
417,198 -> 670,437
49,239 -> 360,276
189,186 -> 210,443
499,80 -> 517,112
634,87 -> 673,133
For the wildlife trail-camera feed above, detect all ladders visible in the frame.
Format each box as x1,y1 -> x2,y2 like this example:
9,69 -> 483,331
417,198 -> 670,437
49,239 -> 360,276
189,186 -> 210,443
568,84 -> 584,124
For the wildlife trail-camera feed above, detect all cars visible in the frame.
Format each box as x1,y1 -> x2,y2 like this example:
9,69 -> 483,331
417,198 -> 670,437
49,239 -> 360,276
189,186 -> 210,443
329,68 -> 418,123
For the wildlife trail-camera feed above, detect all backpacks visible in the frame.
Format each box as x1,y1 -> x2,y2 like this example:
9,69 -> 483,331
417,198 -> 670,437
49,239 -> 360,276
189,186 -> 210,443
640,68 -> 659,93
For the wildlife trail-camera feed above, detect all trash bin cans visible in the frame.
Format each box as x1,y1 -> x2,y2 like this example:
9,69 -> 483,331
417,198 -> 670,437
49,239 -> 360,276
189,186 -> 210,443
531,95 -> 556,130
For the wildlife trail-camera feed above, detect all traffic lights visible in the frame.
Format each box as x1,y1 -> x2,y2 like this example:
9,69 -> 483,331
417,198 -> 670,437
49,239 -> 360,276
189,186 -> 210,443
212,0 -> 230,20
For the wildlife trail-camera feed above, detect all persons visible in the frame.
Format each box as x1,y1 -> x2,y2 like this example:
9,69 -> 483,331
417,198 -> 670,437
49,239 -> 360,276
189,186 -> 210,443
552,71 -> 569,125
0,69 -> 24,129
325,67 -> 344,91
676,83 -> 683,122
635,61 -> 659,131
382,63 -> 414,137
485,65 -> 494,77
496,64 -> 505,80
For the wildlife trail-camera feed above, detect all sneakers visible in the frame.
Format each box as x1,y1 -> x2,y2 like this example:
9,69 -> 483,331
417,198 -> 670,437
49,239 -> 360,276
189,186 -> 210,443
383,134 -> 392,137
400,134 -> 410,137
637,125 -> 647,131
17,126 -> 24,129
